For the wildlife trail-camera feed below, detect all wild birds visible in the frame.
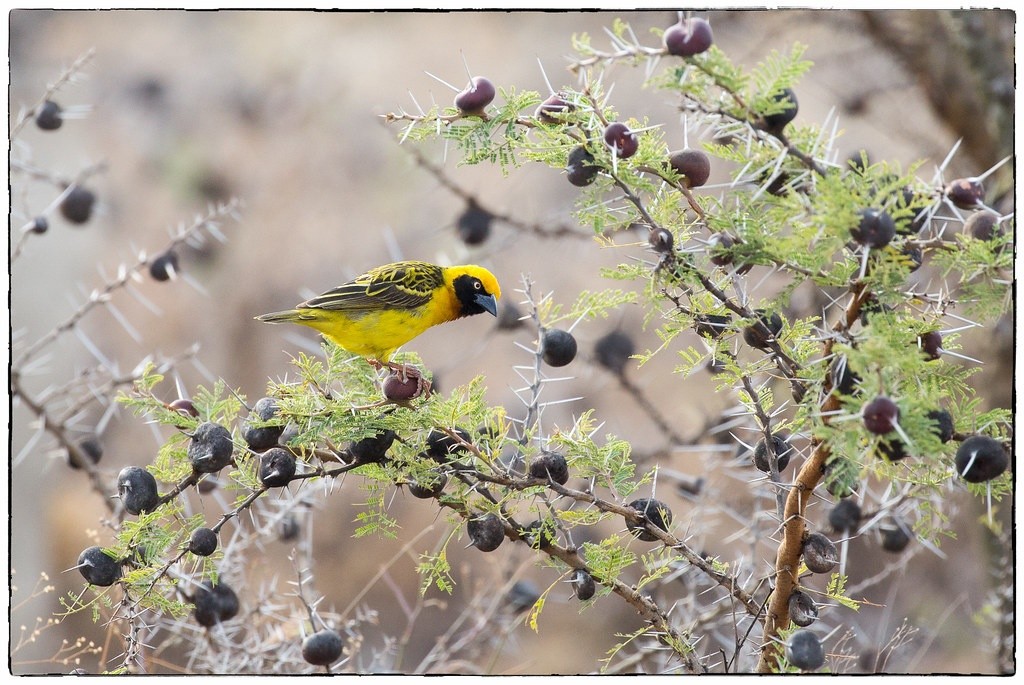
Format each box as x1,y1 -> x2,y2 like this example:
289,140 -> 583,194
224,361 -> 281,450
252,259 -> 502,405
